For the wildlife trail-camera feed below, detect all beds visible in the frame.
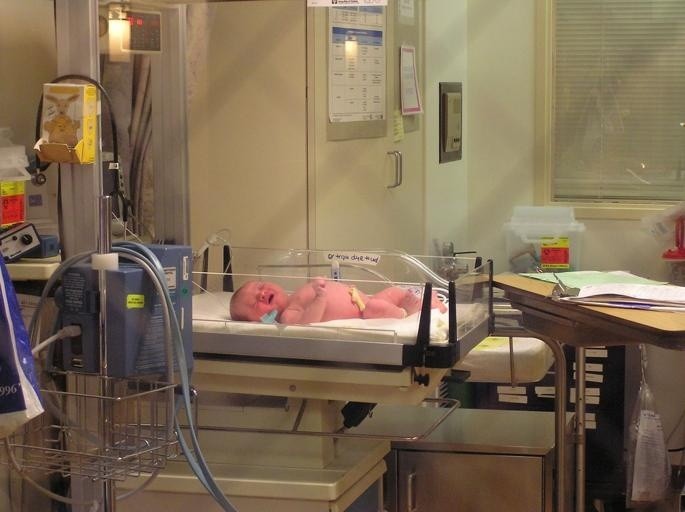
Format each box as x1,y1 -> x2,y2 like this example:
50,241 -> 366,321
190,243 -> 494,364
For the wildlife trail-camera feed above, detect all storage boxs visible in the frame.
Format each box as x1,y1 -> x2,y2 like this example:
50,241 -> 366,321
504,221 -> 587,272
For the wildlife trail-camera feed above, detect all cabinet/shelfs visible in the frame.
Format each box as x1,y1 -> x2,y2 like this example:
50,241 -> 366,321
346,399 -> 575,512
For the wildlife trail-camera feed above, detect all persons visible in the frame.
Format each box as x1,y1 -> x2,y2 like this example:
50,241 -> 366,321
229,280 -> 447,325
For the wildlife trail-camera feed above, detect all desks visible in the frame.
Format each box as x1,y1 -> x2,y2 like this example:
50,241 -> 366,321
494,268 -> 685,512
